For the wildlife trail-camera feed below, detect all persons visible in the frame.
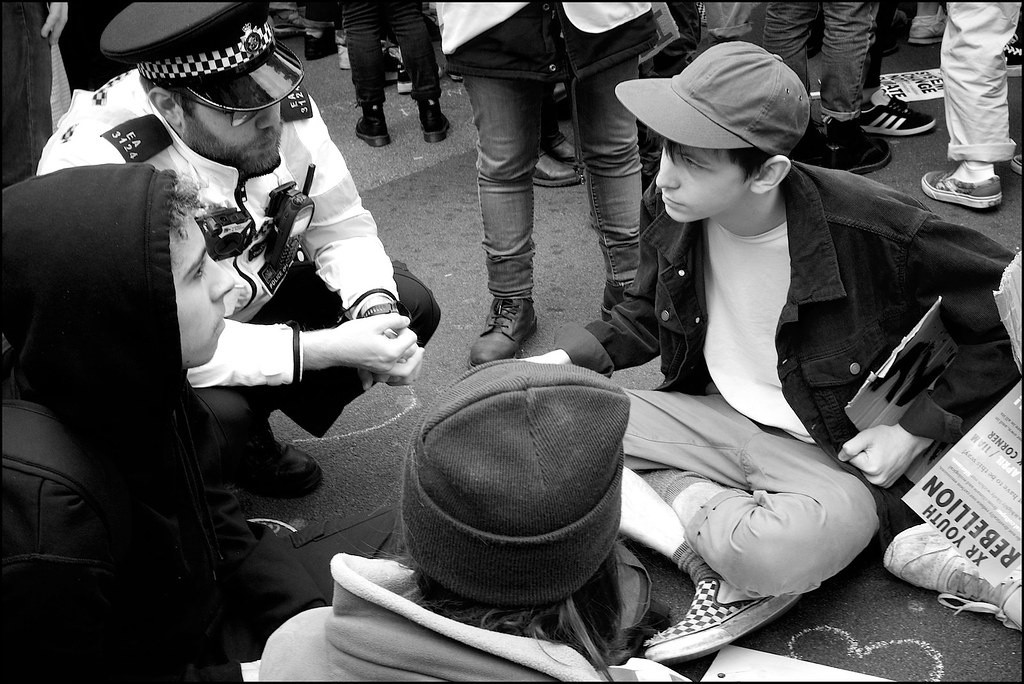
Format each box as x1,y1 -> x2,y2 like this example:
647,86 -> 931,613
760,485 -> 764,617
806,2 -> 1022,208
884,522 -> 1022,632
296,2 -> 413,94
240,358 -> 696,682
1,163 -> 328,683
2,2 -> 69,187
522,42 -> 1019,662
639,2 -> 702,198
36,2 -> 440,497
60,2 -> 139,91
532,83 -> 578,187
765,2 -> 893,175
340,2 -> 450,147
435,2 -> 660,370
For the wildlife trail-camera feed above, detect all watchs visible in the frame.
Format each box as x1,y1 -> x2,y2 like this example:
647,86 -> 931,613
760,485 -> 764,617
363,301 -> 412,320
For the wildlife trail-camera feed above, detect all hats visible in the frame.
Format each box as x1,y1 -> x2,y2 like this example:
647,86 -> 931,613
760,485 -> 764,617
615,40 -> 810,157
400,359 -> 631,606
101,1 -> 306,111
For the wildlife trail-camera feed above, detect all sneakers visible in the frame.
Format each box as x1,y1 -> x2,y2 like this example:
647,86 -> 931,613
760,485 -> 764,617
908,5 -> 947,45
333,39 -> 403,69
857,89 -> 936,136
920,162 -> 1003,209
1010,155 -> 1022,175
397,63 -> 442,93
883,524 -> 1022,633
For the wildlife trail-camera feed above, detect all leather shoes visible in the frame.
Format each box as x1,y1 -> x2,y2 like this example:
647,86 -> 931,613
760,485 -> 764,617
467,295 -> 537,369
601,281 -> 633,322
223,423 -> 322,499
545,136 -> 577,166
303,25 -> 338,61
530,146 -> 580,187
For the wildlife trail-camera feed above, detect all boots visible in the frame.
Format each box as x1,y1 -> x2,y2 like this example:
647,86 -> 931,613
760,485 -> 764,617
415,95 -> 450,144
356,101 -> 391,146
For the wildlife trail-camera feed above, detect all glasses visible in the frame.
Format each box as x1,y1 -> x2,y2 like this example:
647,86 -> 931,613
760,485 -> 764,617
164,88 -> 258,127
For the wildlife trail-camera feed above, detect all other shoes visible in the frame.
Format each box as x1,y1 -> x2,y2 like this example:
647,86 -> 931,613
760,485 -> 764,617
643,576 -> 800,664
882,38 -> 899,57
1003,33 -> 1023,78
787,116 -> 891,174
821,112 -> 868,145
